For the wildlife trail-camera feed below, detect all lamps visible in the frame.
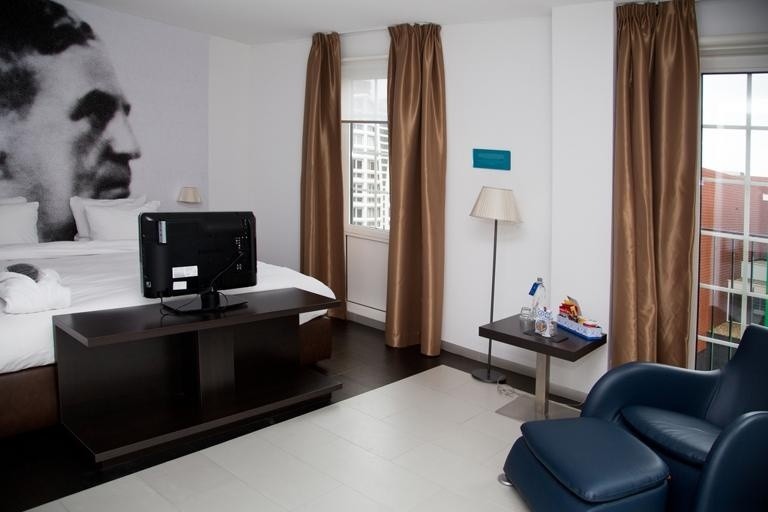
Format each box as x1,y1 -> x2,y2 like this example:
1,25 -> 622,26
469,187 -> 525,385
177,186 -> 201,204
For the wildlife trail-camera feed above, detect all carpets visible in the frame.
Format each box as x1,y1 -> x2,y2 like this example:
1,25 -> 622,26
0,348 -> 581,512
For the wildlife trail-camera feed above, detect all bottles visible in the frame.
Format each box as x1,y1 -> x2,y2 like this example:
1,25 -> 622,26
520,307 -> 533,332
530,278 -> 546,321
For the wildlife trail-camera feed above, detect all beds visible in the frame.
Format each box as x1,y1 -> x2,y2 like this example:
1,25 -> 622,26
0,236 -> 337,376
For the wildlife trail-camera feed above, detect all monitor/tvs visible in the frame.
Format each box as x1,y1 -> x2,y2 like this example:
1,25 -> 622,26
138,210 -> 257,313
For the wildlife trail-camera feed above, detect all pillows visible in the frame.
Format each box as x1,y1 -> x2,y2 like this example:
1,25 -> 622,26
82,202 -> 160,241
71,195 -> 146,241
0,202 -> 40,245
1,197 -> 27,206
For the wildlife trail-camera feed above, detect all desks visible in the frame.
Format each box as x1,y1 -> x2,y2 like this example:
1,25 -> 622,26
477,308 -> 608,430
51,287 -> 343,469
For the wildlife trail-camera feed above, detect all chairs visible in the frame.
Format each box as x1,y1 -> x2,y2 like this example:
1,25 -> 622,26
502,323 -> 767,511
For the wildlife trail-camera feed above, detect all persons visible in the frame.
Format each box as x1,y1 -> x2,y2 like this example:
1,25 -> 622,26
0,0 -> 142,243
